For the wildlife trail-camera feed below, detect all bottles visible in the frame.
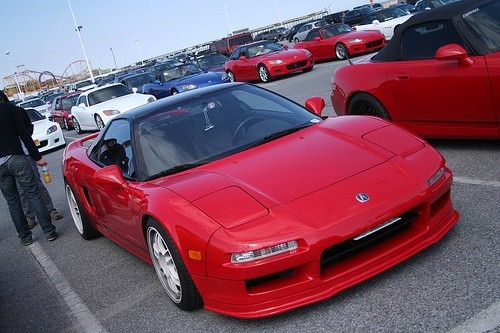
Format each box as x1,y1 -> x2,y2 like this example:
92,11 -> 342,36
42,165 -> 52,183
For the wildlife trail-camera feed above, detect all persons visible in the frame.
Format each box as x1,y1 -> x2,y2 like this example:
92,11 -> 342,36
0,89 -> 59,246
2,116 -> 63,231
95,137 -> 132,173
255,45 -> 271,56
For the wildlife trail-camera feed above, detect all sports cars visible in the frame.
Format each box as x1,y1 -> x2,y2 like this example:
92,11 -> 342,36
142,63 -> 232,99
61,81 -> 460,319
351,6 -> 420,42
330,0 -> 500,141
224,40 -> 314,84
292,22 -> 387,63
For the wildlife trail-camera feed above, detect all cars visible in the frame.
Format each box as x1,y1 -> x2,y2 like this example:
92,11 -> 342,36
49,91 -> 90,132
16,107 -> 66,156
70,83 -> 157,135
16,97 -> 54,122
15,3 -> 385,105
390,0 -> 462,13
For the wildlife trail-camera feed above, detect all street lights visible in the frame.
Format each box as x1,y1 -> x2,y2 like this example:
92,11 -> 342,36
5,50 -> 25,102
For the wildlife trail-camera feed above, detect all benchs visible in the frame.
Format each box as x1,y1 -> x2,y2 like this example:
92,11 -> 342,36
402,20 -> 472,58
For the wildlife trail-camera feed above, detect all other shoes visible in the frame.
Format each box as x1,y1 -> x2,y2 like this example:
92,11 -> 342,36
47,232 -> 58,240
50,209 -> 63,220
20,238 -> 33,246
28,216 -> 36,229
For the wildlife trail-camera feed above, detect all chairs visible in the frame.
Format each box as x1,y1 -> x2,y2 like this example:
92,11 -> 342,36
137,127 -> 194,177
199,102 -> 250,155
